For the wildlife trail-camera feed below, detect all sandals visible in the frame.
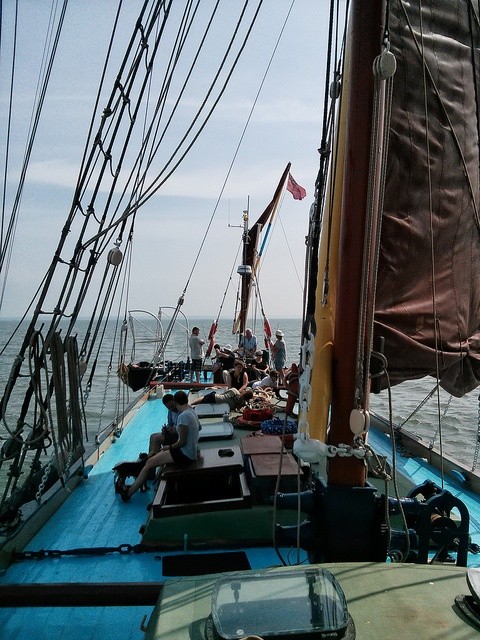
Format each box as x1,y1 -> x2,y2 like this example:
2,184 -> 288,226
117,477 -> 131,502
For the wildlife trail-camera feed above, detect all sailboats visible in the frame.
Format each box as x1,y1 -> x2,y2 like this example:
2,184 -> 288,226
0,0 -> 479,639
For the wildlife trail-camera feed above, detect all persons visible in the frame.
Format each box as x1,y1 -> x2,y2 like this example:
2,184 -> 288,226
149,393 -> 183,455
267,330 -> 288,386
188,388 -> 252,411
211,344 -> 223,362
250,350 -> 269,380
252,370 -> 278,392
227,360 -> 249,392
116,391 -> 200,503
238,328 -> 257,358
189,326 -> 205,383
215,344 -> 235,370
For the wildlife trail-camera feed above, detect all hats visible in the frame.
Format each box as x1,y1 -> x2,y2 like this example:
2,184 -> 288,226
223,344 -> 233,352
274,330 -> 284,336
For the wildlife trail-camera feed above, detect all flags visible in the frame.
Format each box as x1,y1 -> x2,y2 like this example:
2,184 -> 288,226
286,173 -> 306,200
